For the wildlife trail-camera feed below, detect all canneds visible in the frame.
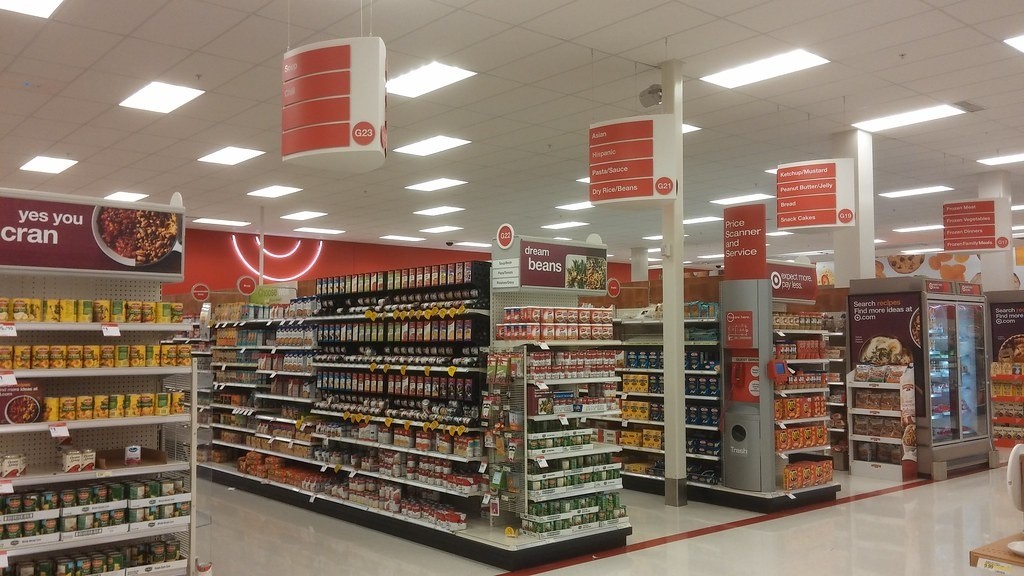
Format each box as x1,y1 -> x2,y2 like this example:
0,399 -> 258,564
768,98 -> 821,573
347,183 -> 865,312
0,475 -> 190,576
554,383 -> 620,415
316,260 -> 471,295
521,435 -> 627,533
0,296 -> 191,422
526,349 -> 616,379
771,310 -> 829,390
302,422 -> 489,531
496,305 -> 614,341
0,443 -> 141,478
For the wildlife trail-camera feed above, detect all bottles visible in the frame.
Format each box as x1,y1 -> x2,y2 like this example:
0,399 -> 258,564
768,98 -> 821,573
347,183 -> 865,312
775,339 -> 824,360
772,312 -> 823,330
787,369 -> 827,389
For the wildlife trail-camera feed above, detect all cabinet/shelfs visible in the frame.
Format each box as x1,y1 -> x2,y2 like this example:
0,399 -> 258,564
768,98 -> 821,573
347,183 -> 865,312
0,186 -> 197,576
818,328 -> 918,481
990,373 -> 1024,448
577,262 -> 842,515
183,234 -> 634,571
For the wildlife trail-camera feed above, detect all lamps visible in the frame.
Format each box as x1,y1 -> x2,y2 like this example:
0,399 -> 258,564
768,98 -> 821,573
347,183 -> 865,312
0,1 -> 1023,269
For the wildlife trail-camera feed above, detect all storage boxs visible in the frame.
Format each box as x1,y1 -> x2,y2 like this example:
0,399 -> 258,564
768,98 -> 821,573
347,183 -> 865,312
990,361 -> 1024,439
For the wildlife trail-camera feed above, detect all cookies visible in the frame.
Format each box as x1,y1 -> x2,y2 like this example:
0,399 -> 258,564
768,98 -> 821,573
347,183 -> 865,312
875,254 -> 925,278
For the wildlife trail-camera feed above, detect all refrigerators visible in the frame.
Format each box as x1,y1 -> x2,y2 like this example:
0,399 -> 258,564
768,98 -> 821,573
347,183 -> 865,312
847,279 -> 993,474
